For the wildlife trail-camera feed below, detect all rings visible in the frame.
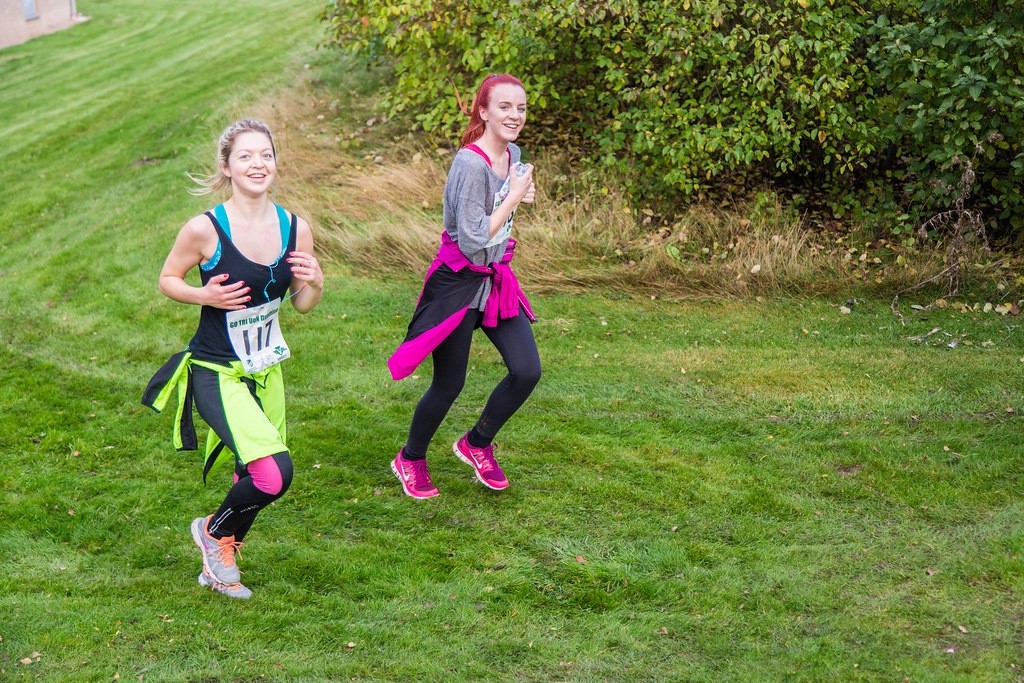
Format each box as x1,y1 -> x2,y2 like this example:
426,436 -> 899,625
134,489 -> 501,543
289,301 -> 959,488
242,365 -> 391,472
306,260 -> 311,269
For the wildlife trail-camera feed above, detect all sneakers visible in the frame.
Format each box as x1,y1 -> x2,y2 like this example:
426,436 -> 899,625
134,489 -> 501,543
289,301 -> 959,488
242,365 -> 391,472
454,431 -> 510,490
191,514 -> 243,586
197,571 -> 254,600
390,448 -> 440,499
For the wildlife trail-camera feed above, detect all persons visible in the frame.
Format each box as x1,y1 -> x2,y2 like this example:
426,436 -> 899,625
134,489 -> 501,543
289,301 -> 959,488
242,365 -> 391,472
382,72 -> 542,499
156,121 -> 325,603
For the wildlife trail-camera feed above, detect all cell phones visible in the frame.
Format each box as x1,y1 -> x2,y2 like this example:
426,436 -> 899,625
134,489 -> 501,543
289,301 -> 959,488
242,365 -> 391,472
499,162 -> 528,200
